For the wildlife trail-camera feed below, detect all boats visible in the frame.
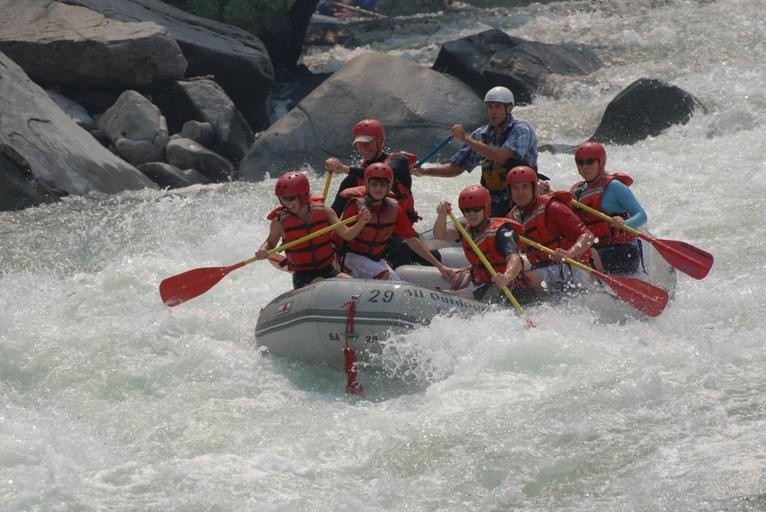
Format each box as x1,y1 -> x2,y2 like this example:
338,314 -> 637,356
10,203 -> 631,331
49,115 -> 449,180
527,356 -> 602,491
254,214 -> 677,378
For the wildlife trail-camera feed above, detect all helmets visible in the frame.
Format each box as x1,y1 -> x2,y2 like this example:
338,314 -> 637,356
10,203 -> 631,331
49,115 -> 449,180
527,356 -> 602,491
353,119 -> 393,192
458,185 -> 492,217
484,86 -> 514,107
505,166 -> 537,199
275,171 -> 310,206
575,142 -> 606,167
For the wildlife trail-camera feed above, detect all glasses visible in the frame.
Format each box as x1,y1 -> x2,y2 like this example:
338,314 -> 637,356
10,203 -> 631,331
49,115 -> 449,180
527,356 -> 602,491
577,160 -> 595,165
279,196 -> 296,201
463,208 -> 483,212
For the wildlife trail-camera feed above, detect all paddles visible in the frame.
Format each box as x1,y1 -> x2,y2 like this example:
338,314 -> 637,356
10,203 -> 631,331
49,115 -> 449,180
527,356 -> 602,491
159,212 -> 367,307
517,235 -> 668,316
547,188 -> 713,280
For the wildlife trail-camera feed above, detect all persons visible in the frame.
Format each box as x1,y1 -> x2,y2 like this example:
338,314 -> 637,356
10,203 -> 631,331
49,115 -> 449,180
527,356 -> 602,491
568,141 -> 647,276
322,119 -> 437,267
410,86 -> 538,219
258,172 -> 370,291
501,165 -> 595,293
433,185 -> 535,308
341,162 -> 453,286
